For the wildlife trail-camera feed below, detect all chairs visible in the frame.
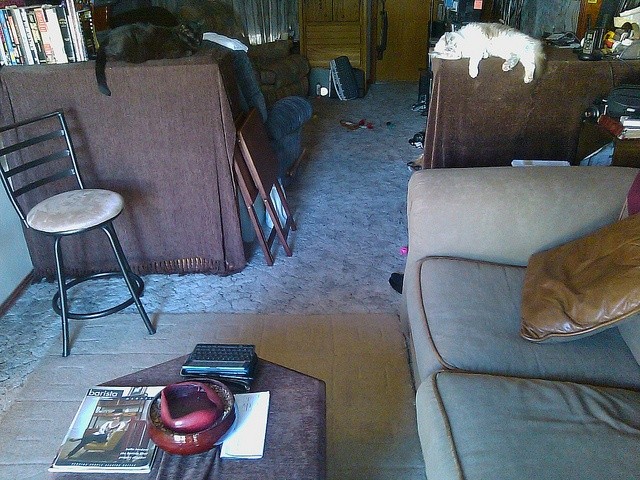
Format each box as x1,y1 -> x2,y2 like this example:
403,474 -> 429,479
0,109 -> 156,358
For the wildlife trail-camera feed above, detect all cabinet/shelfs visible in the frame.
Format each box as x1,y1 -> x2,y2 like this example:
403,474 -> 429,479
299,0 -> 370,92
278,39 -> 299,53
576,119 -> 640,167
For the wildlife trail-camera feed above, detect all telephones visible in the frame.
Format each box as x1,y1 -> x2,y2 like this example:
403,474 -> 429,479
578,29 -> 602,61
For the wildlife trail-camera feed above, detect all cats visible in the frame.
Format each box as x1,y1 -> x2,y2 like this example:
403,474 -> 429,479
428,20 -> 547,84
95,14 -> 206,96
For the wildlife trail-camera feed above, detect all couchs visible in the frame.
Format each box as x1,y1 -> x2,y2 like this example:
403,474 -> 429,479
250,41 -> 311,99
202,32 -> 312,241
399,167 -> 640,480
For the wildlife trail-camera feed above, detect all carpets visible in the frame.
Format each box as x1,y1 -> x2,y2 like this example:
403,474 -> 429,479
0,312 -> 426,480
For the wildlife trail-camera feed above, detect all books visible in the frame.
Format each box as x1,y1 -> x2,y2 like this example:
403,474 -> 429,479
48,385 -> 168,474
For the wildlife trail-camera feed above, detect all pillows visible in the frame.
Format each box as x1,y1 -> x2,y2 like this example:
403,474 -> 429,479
519,213 -> 639,342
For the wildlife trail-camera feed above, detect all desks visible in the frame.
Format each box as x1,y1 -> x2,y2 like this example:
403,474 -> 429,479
50,354 -> 326,480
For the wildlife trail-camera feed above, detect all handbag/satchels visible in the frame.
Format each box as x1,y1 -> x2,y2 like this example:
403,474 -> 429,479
602,86 -> 640,118
